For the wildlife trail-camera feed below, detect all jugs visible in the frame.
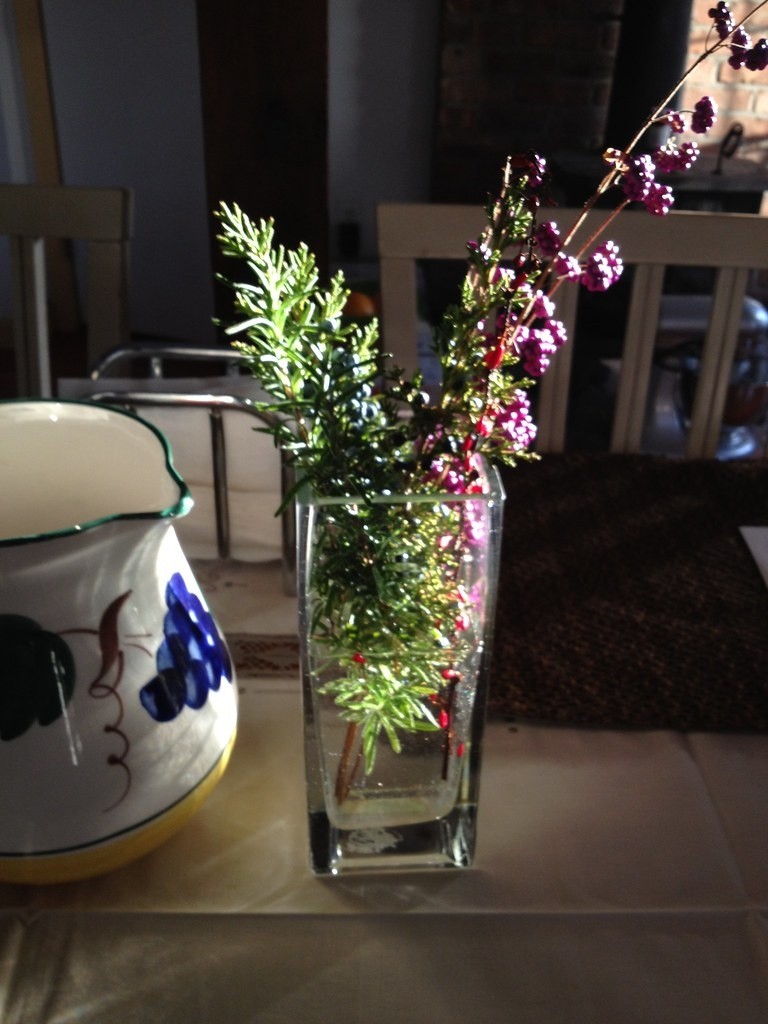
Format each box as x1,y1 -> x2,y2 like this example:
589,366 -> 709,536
0,398 -> 236,884
